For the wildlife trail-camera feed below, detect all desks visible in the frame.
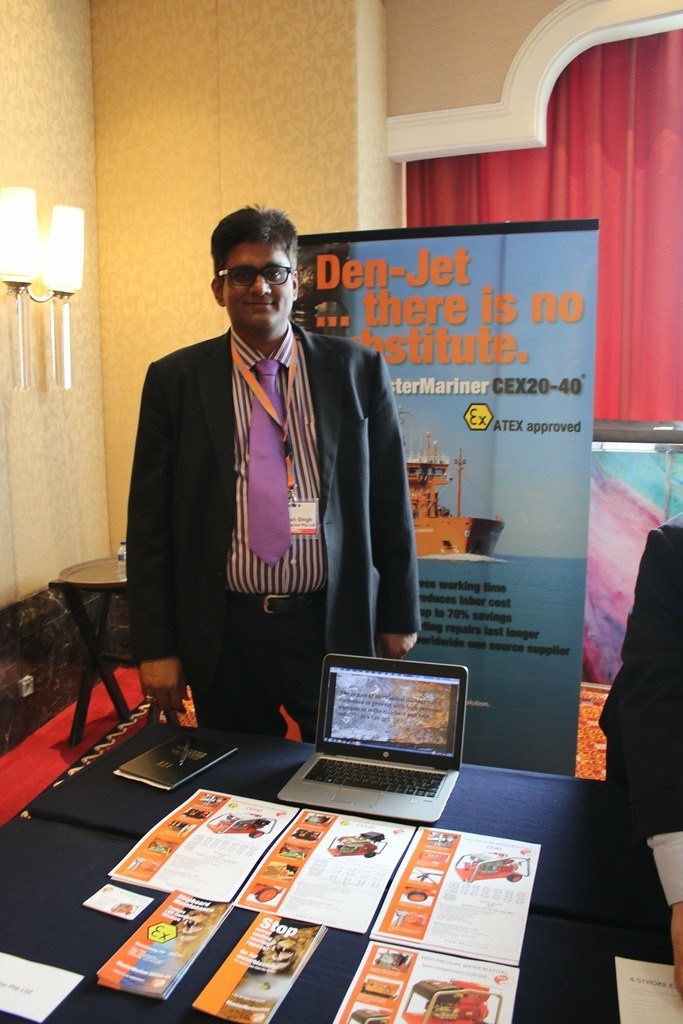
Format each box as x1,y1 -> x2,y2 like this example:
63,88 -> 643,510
49,555 -> 190,746
0,722 -> 683,1024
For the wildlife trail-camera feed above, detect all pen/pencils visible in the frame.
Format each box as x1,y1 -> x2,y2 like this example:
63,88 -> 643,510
178,737 -> 192,767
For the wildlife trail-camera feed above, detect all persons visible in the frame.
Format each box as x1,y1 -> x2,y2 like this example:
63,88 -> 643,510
125,207 -> 423,751
592,507 -> 682,1000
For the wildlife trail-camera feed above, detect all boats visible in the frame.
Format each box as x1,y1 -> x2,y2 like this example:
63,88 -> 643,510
406,433 -> 504,556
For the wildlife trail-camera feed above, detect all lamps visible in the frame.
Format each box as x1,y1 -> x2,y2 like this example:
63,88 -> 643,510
0,187 -> 84,390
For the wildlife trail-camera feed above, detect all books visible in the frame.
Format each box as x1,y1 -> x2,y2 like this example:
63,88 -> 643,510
117,733 -> 240,791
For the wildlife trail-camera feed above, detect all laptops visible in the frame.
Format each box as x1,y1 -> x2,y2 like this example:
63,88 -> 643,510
277,654 -> 469,823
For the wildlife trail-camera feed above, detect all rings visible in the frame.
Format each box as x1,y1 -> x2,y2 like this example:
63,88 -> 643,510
145,695 -> 159,705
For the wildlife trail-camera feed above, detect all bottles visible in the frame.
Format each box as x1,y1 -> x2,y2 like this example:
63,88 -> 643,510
117,541 -> 127,582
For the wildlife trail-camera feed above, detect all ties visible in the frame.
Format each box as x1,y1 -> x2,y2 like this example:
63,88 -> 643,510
248,360 -> 294,568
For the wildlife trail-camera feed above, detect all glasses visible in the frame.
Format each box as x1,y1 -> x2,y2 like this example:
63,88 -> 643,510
219,264 -> 294,286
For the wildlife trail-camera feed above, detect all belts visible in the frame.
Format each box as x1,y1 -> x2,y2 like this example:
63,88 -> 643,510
228,590 -> 326,615
